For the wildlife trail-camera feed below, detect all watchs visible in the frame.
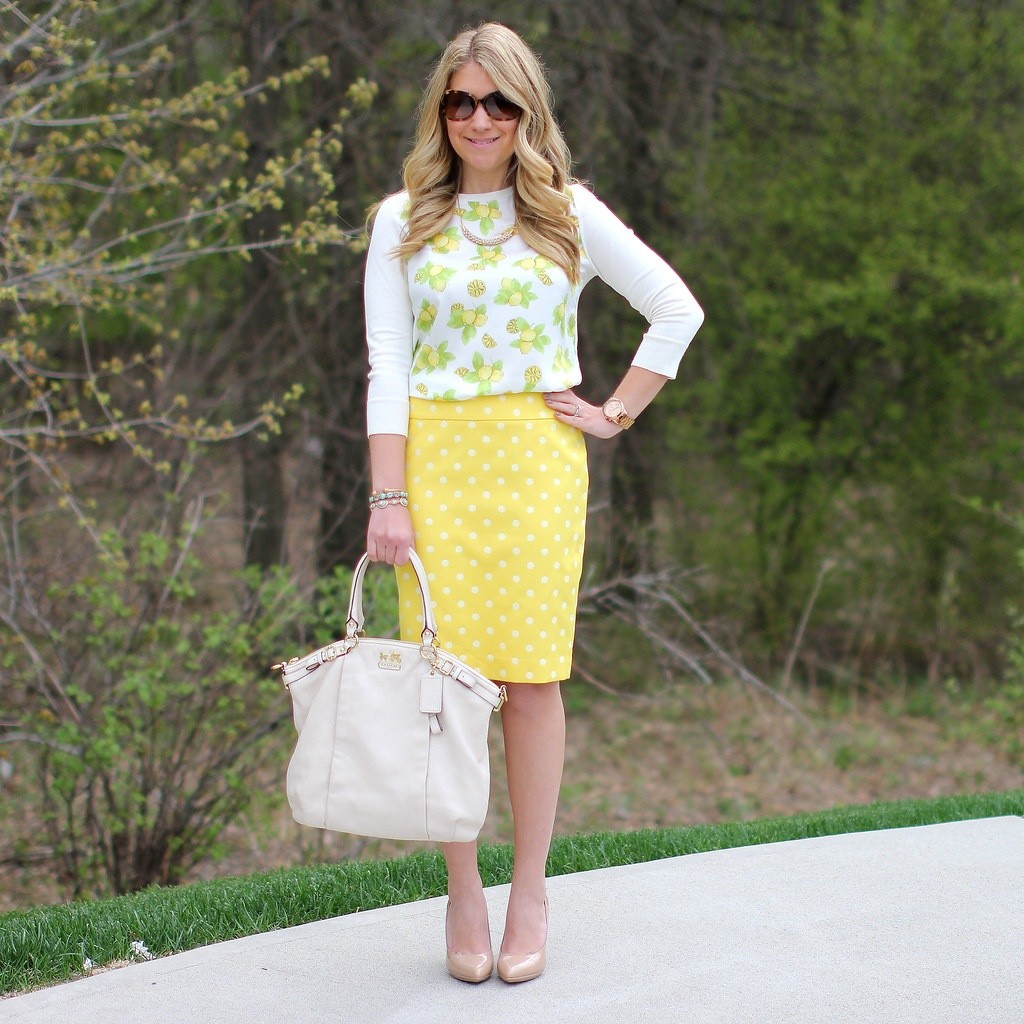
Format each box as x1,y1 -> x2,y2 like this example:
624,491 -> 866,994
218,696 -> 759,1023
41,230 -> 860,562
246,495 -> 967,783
601,398 -> 634,430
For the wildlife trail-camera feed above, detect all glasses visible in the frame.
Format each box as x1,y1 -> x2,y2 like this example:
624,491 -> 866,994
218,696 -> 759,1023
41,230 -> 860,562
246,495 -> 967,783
440,90 -> 523,121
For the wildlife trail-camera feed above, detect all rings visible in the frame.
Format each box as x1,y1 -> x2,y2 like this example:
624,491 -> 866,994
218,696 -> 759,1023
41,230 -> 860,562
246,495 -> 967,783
575,406 -> 580,416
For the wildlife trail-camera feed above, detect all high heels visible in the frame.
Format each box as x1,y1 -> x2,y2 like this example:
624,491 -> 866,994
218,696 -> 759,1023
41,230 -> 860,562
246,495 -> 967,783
497,894 -> 549,982
445,899 -> 494,982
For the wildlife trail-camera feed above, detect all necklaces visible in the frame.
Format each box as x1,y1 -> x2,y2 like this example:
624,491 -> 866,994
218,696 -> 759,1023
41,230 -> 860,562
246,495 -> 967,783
457,194 -> 514,247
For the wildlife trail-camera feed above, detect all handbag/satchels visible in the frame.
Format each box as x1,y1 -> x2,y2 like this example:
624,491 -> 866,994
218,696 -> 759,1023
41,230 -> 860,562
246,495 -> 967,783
271,547 -> 508,843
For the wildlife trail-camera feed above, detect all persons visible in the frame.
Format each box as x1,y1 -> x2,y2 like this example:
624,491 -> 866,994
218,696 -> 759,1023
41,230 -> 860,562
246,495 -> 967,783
365,23 -> 705,984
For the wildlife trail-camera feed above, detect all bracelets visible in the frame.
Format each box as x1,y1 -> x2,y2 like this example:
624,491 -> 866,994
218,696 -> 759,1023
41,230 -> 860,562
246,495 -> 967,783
369,489 -> 407,509
